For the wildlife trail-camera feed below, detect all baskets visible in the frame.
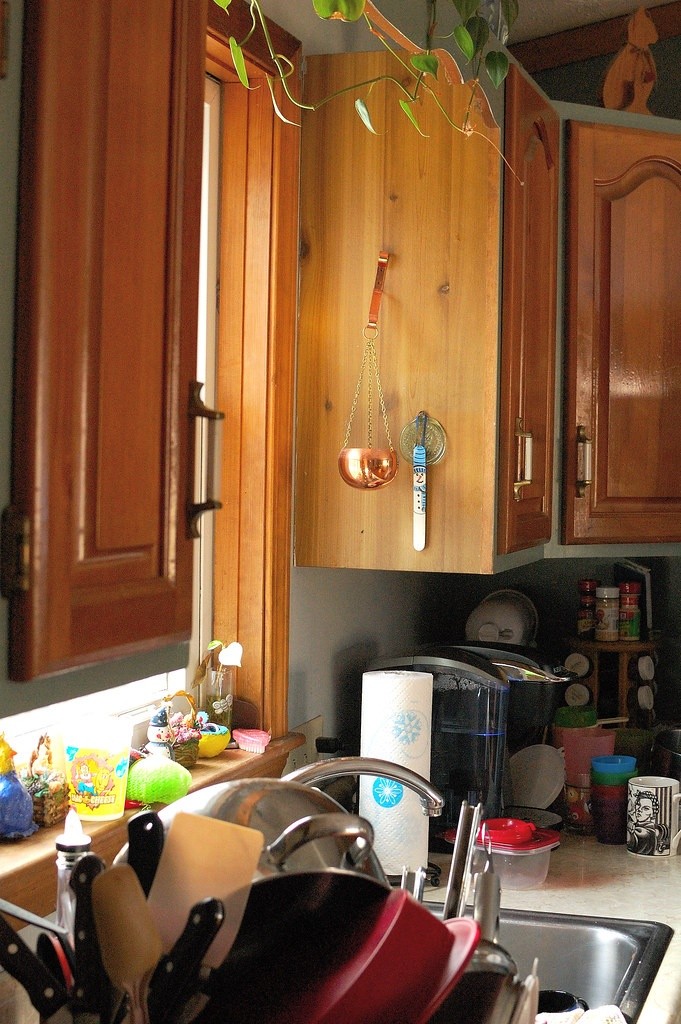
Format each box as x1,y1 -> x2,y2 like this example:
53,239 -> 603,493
161,690 -> 202,768
18,735 -> 71,828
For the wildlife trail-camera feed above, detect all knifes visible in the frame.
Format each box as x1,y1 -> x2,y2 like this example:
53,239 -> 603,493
0,812 -> 265,1024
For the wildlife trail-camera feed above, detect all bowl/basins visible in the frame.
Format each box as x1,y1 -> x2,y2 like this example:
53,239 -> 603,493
444,827 -> 562,891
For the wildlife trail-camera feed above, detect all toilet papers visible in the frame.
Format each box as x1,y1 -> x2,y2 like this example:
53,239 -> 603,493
359,669 -> 434,876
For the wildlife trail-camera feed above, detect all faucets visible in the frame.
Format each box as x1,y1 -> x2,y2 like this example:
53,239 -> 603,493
280,755 -> 446,818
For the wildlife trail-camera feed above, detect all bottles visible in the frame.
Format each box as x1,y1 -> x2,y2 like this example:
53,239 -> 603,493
574,579 -> 642,642
559,651 -> 659,720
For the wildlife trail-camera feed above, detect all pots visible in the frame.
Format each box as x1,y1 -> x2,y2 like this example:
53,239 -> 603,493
108,778 -> 397,1006
466,588 -> 539,646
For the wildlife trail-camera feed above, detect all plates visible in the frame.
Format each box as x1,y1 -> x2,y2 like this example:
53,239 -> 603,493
506,744 -> 566,810
505,807 -> 563,829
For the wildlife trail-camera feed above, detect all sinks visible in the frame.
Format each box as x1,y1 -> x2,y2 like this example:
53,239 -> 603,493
419,897 -> 675,1024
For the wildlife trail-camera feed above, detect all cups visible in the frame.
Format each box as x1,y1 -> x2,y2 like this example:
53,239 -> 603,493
550,705 -> 681,858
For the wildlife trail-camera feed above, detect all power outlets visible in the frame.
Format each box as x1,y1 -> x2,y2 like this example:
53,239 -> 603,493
281,716 -> 322,776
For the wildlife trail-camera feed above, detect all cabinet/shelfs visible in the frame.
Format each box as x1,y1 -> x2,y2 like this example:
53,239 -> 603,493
562,103 -> 681,546
499,54 -> 552,556
8,2 -> 209,683
